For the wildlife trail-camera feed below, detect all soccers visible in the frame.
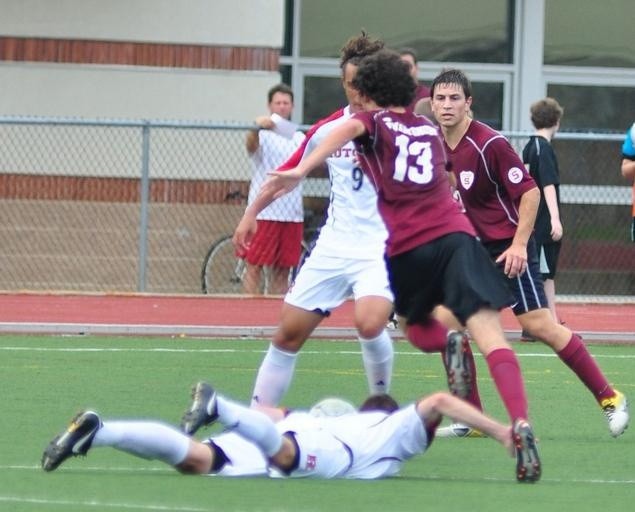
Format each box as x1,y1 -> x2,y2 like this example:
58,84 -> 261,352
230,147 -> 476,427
314,400 -> 356,416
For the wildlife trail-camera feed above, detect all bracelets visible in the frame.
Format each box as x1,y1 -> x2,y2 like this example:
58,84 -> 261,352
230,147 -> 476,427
249,119 -> 260,131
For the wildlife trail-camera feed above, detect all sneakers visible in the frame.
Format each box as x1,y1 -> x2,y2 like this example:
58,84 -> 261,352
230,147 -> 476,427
41,409 -> 104,471
180,381 -> 221,437
447,330 -> 473,399
601,388 -> 630,438
435,423 -> 488,437
519,328 -> 541,342
512,420 -> 542,483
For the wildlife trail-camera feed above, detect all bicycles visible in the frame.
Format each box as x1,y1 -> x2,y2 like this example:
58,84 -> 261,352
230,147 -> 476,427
199,189 -> 323,296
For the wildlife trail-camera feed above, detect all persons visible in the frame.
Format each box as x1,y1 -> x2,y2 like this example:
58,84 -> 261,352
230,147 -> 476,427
243,84 -> 309,293
520,99 -> 581,344
397,48 -> 432,117
620,122 -> 635,277
428,69 -> 628,438
259,48 -> 542,482
232,27 -> 398,404
39,379 -> 513,477
